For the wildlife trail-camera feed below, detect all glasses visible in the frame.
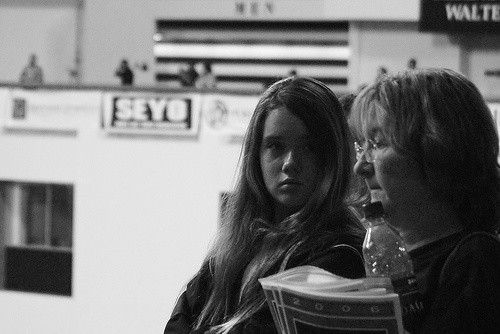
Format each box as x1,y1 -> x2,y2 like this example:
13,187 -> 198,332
354,141 -> 400,163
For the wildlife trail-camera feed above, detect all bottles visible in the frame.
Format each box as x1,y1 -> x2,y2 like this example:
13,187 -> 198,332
361,201 -> 423,317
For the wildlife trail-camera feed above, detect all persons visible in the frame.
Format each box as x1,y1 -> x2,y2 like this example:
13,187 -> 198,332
164,75 -> 368,334
348,67 -> 500,334
